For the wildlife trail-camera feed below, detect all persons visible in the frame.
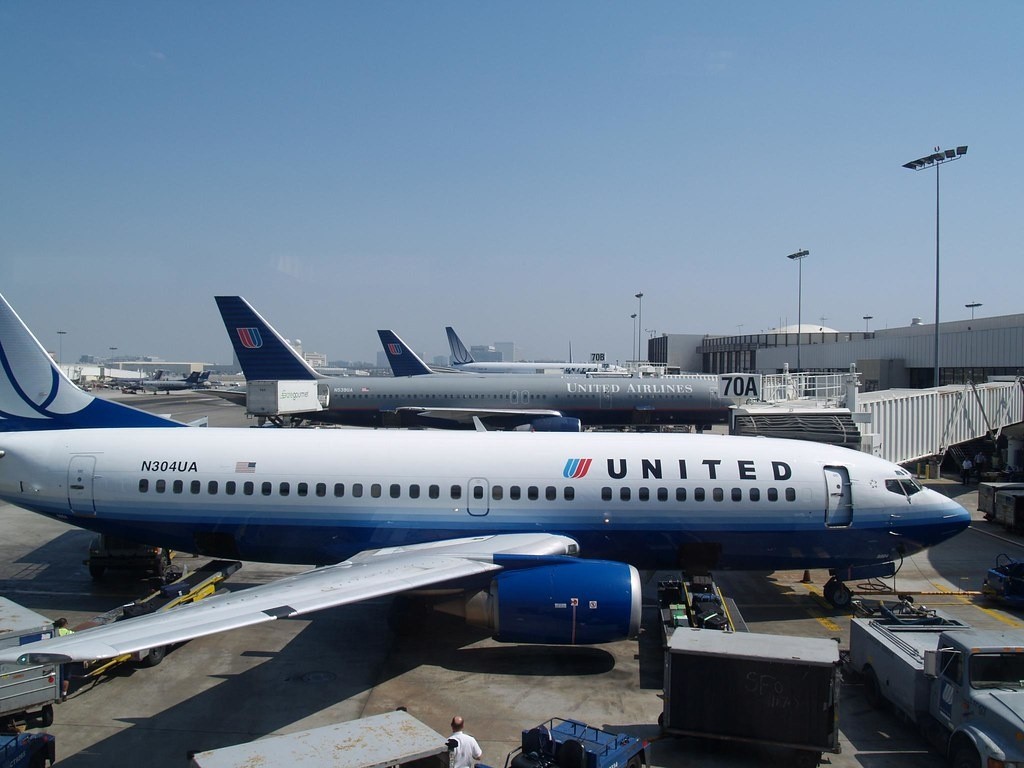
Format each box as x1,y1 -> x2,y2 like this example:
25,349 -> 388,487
56,618 -> 76,702
961,457 -> 973,484
990,452 -> 1000,471
446,716 -> 482,768
974,452 -> 986,478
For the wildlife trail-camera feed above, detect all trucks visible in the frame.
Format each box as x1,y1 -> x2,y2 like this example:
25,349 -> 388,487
843,608 -> 1024,768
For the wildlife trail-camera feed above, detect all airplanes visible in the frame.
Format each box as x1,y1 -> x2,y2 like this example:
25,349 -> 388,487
213,294 -> 763,432
0,294 -> 972,668
138,370 -> 213,394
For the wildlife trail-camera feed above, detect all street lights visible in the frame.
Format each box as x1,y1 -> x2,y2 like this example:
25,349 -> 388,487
630,291 -> 644,361
786,248 -> 809,374
901,145 -> 968,389
108,347 -> 117,363
964,302 -> 983,317
860,314 -> 874,330
56,331 -> 66,370
